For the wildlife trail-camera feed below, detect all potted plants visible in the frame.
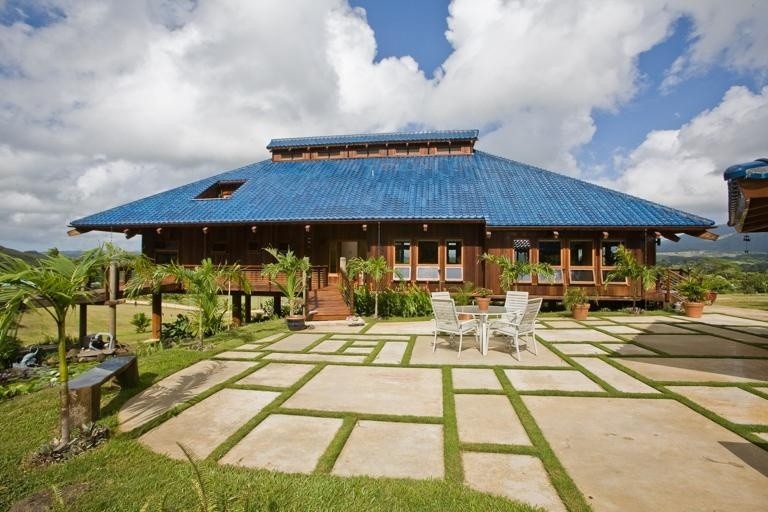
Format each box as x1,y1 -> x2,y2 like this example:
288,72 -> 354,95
472,286 -> 494,309
672,281 -> 710,316
563,286 -> 590,320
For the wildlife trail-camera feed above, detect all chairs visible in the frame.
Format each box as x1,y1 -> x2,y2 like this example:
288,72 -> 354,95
486,297 -> 544,359
431,292 -> 452,301
431,298 -> 480,360
490,290 -> 528,352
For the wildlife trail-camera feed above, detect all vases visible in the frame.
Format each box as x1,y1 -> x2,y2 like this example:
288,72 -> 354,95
285,315 -> 306,330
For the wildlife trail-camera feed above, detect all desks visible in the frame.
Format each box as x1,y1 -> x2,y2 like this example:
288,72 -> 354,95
453,305 -> 521,356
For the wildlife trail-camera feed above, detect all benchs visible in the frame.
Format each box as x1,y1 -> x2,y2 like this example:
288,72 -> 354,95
64,355 -> 139,424
